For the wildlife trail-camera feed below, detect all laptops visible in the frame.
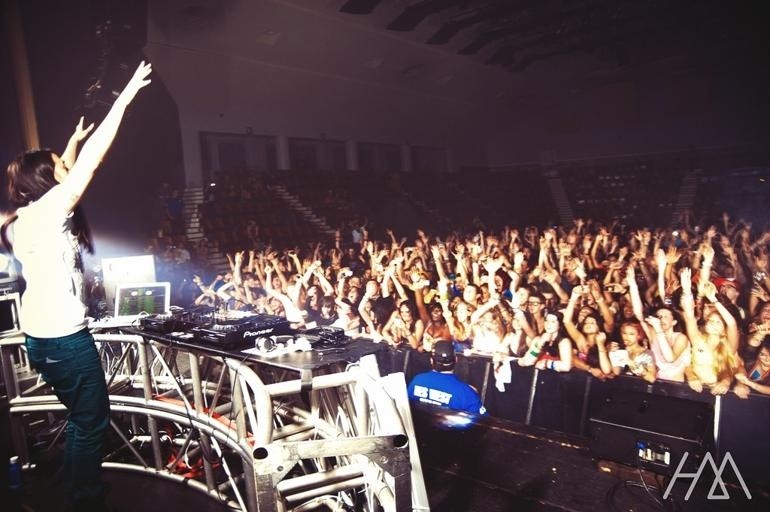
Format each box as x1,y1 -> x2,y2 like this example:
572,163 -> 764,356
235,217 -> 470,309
87,282 -> 172,330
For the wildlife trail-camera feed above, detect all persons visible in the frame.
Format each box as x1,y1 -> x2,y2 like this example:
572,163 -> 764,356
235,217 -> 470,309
139,169 -> 770,423
0,59 -> 153,510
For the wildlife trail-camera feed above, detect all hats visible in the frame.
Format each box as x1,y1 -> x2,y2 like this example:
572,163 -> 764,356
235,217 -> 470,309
435,340 -> 454,364
713,277 -> 740,289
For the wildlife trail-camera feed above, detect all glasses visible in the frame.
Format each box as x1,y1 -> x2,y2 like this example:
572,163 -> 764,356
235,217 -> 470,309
719,283 -> 738,290
528,302 -> 545,306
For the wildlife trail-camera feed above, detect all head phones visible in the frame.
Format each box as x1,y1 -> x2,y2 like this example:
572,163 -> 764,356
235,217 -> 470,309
254,335 -> 299,352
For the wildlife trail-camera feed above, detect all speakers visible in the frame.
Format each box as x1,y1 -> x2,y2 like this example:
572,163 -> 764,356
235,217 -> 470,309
583,397 -> 714,482
